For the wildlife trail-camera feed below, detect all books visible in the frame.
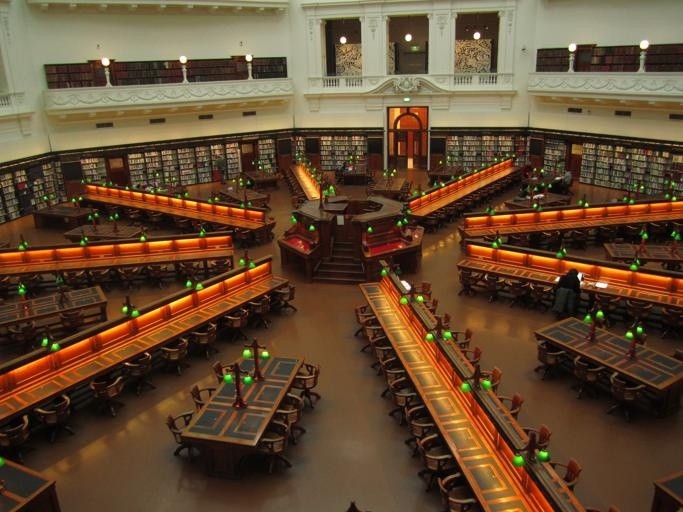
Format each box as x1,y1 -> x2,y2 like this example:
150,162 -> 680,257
128,142 -> 242,192
0,156 -> 106,225
446,134 -> 567,175
579,141 -> 683,199
321,135 -> 368,171
258,136 -> 306,176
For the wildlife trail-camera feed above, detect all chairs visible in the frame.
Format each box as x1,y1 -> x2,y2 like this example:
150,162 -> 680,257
292,358 -> 322,409
165,410 -> 212,462
355,222 -> 682,512
274,392 -> 307,436
258,420 -> 293,473
1,204 -> 298,438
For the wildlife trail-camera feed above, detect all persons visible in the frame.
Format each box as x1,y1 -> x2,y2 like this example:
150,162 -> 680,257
552,267 -> 582,317
559,167 -> 572,194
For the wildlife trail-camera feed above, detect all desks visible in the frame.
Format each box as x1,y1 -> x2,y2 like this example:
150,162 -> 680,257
181,354 -> 306,447
